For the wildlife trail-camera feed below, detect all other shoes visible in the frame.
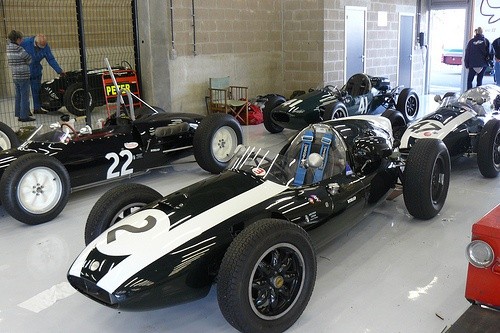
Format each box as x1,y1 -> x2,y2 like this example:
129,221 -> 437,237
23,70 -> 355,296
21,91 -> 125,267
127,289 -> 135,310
18,117 -> 36,122
34,109 -> 47,114
28,111 -> 32,116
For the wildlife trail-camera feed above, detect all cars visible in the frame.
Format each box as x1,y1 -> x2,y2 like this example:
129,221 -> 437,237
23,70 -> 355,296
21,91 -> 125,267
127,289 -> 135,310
66,114 -> 453,333
40,61 -> 138,118
262,72 -> 421,135
0,88 -> 244,225
382,82 -> 500,179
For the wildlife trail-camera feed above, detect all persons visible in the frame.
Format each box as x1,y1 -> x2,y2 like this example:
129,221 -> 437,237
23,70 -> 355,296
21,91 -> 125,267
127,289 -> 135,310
492,37 -> 500,87
464,27 -> 495,91
20,33 -> 66,117
7,30 -> 36,122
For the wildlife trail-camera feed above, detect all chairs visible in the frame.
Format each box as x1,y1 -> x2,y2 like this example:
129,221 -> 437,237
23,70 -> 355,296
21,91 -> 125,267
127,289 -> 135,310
283,124 -> 347,181
208,76 -> 249,125
346,73 -> 372,96
